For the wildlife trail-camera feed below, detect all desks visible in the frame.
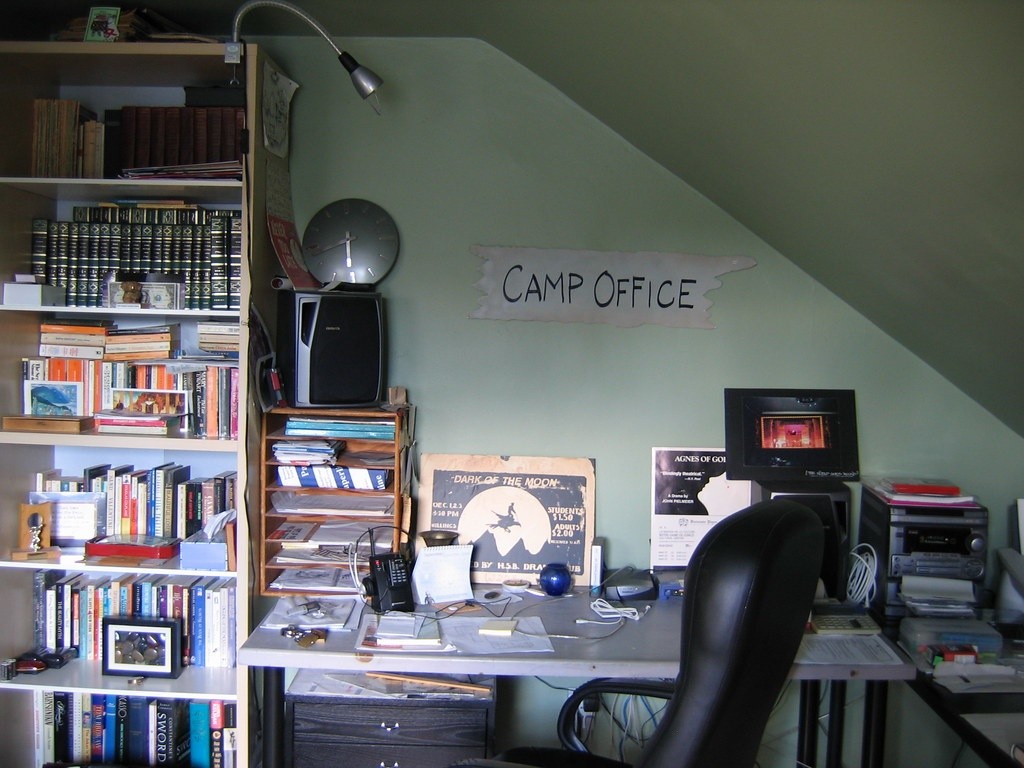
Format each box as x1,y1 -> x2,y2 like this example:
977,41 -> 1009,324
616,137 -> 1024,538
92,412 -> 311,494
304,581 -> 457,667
237,570 -> 917,768
862,610 -> 1024,768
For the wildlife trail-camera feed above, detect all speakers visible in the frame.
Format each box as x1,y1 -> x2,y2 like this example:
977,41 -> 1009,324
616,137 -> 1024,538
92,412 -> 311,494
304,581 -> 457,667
276,291 -> 386,410
750,481 -> 852,606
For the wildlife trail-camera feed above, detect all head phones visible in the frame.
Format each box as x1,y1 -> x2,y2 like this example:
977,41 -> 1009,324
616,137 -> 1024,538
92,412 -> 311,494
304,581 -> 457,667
354,526 -> 416,597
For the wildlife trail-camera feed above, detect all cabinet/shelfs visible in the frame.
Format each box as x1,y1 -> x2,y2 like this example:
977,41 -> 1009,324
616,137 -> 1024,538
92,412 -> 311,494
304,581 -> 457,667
260,402 -> 413,597
282,669 -> 499,768
0,42 -> 288,768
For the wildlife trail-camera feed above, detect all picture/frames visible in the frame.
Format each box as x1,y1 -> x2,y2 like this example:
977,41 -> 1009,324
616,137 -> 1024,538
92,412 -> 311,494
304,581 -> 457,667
724,387 -> 860,482
102,615 -> 183,680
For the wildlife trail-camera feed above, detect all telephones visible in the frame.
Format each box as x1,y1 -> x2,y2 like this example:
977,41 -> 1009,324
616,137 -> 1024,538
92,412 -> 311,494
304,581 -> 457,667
367,527 -> 416,612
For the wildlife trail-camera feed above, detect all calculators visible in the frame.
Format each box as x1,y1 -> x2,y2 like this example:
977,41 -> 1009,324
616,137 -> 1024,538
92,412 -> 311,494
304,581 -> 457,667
810,605 -> 882,634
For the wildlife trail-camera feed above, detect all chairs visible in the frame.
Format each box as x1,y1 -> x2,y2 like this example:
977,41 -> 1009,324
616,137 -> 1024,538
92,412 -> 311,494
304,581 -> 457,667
445,500 -> 825,768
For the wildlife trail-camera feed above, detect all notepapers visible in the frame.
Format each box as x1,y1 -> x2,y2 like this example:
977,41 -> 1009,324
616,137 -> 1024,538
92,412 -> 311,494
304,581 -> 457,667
476,620 -> 518,636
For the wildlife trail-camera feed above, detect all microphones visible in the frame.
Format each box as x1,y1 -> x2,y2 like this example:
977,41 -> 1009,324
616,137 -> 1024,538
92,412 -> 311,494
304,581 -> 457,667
348,543 -> 364,596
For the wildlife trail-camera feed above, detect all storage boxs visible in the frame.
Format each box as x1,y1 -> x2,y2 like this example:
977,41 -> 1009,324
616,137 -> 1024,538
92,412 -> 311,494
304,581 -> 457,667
898,617 -> 1004,655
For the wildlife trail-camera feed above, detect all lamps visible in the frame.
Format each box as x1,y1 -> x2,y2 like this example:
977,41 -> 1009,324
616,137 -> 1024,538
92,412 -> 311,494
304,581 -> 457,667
224,0 -> 388,116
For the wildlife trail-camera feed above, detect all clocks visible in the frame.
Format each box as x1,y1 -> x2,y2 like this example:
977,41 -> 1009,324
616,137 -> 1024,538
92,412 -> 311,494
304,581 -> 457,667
302,198 -> 400,284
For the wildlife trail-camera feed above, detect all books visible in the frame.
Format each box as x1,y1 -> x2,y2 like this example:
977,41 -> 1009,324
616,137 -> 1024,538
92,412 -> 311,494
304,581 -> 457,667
34,690 -> 237,768
1,319 -> 239,441
33,99 -> 248,181
16,460 -> 238,669
263,415 -> 458,654
16,198 -> 242,311
